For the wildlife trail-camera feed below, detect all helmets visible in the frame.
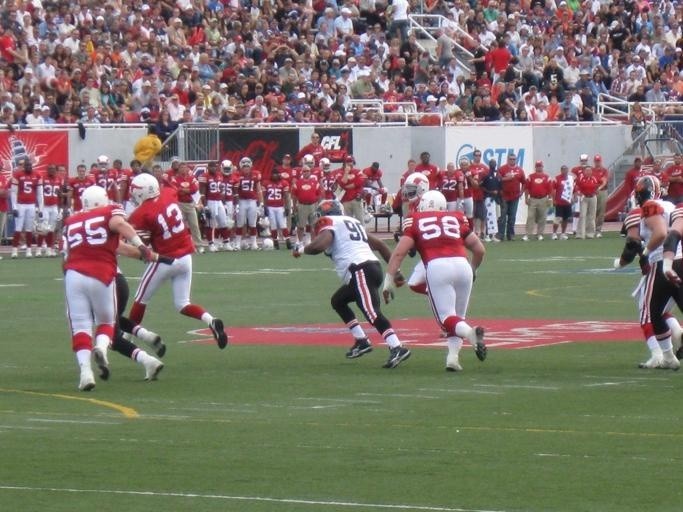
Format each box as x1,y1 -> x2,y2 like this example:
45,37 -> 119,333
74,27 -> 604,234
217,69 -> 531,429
404,172 -> 430,203
221,159 -> 234,176
458,155 -> 471,168
315,199 -> 345,216
169,155 -> 182,170
418,190 -> 449,213
130,172 -> 160,204
319,157 -> 333,174
238,156 -> 252,169
580,154 -> 591,168
96,155 -> 111,169
82,186 -> 109,210
629,174 -> 661,207
302,153 -> 315,168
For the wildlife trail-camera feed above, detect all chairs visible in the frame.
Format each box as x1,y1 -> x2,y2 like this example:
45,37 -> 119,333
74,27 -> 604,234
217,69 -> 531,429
364,194 -> 402,234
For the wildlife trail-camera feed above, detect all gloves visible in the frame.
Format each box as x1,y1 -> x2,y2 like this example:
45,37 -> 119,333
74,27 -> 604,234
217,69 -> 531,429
290,248 -> 305,258
662,258 -> 682,290
613,257 -> 623,270
157,253 -> 183,266
639,254 -> 651,276
393,269 -> 407,287
137,245 -> 152,264
381,273 -> 394,306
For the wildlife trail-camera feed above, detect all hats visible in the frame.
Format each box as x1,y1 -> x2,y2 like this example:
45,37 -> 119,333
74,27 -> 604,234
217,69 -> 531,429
21,0 -> 332,72
578,69 -> 591,75
297,92 -> 306,99
348,57 -> 356,63
170,93 -> 180,100
427,93 -> 458,101
332,58 -> 341,65
594,154 -> 602,161
141,80 -> 152,87
2,91 -> 13,98
344,155 -> 353,162
489,1 -> 683,64
219,82 -> 228,90
208,161 -> 218,167
32,103 -> 50,112
535,160 -> 544,168
341,7 -> 353,13
202,84 -> 211,90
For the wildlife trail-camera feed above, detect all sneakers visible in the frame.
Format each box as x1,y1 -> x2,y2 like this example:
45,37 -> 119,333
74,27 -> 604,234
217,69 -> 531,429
446,355 -> 464,371
144,357 -> 164,381
382,346 -> 411,369
208,317 -> 228,349
10,246 -> 58,259
467,325 -> 487,361
671,326 -> 683,360
142,331 -> 166,357
345,336 -> 373,358
78,369 -> 96,391
194,238 -> 311,253
91,344 -> 110,381
638,354 -> 681,370
480,232 -> 605,243
